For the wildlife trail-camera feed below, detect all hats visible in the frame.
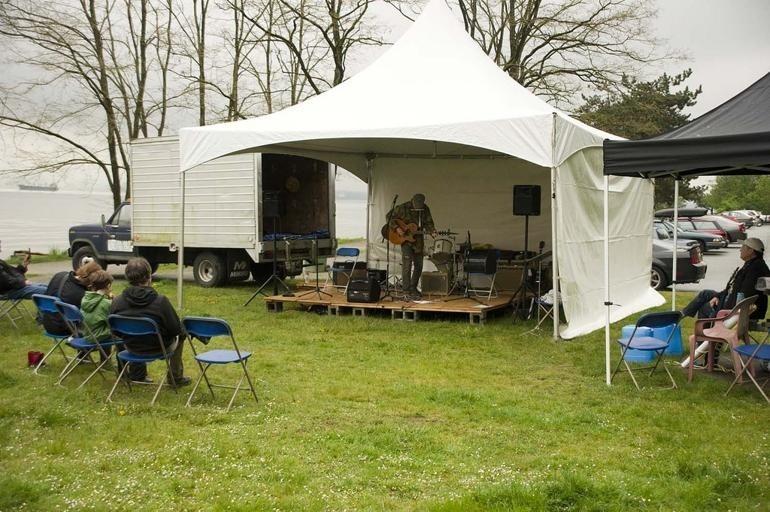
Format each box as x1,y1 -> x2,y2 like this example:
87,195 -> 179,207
413,194 -> 425,209
737,238 -> 764,253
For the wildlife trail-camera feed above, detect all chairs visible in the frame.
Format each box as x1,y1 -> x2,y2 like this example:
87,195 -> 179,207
689,295 -> 758,385
33,293 -> 106,376
105,314 -> 179,406
181,317 -> 258,414
323,248 -> 360,295
464,244 -> 502,300
610,311 -> 681,393
55,300 -> 128,392
723,331 -> 770,403
0,294 -> 26,329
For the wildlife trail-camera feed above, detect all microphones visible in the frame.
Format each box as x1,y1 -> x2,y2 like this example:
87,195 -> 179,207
392,191 -> 399,206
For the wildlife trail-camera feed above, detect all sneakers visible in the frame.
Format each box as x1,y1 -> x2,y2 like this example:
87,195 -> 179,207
102,364 -> 118,371
169,376 -> 192,386
410,290 -> 422,297
141,378 -> 154,386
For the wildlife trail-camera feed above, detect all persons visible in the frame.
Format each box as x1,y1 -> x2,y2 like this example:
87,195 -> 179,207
0,239 -> 49,329
110,257 -> 192,387
679,238 -> 770,368
386,194 -> 438,297
41,263 -> 102,364
79,269 -> 129,378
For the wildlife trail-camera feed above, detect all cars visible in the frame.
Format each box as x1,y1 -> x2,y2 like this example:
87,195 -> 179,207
646,206 -> 769,293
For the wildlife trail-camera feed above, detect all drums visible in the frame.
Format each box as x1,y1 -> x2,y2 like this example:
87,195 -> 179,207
433,240 -> 452,261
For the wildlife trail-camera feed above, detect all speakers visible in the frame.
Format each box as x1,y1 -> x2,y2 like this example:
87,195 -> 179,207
513,184 -> 541,216
347,277 -> 380,304
262,190 -> 287,219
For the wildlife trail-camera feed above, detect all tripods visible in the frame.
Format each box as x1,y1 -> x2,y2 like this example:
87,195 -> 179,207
241,222 -> 292,308
380,243 -> 404,303
507,217 -> 540,324
298,240 -> 333,302
445,262 -> 468,298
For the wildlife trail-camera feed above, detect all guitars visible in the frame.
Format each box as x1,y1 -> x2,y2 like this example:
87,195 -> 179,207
388,218 -> 437,244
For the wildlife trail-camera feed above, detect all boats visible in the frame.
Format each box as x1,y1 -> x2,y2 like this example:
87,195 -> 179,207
17,184 -> 59,192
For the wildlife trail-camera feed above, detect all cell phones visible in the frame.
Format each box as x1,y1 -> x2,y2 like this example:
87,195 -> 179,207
27,248 -> 31,259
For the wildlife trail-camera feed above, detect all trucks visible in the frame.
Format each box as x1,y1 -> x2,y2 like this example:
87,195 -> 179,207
68,134 -> 340,285
